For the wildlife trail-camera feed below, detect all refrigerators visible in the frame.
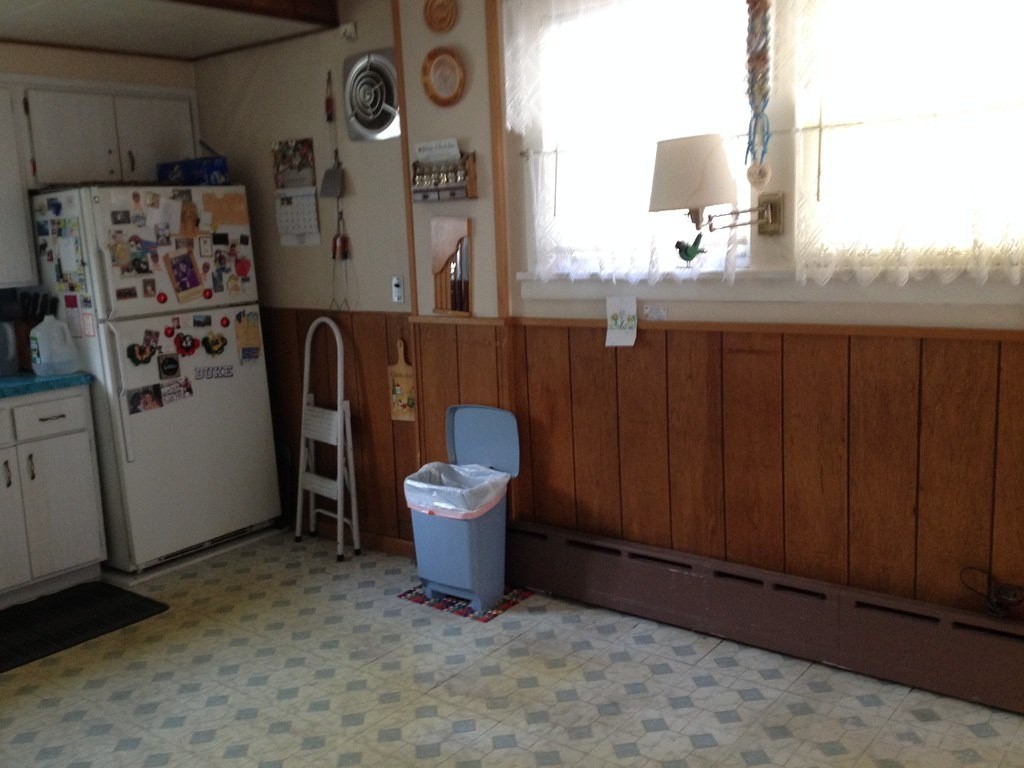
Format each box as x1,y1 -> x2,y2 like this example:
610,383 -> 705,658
27,176 -> 288,587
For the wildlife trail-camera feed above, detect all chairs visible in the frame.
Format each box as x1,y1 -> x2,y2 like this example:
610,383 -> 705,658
295,315 -> 361,562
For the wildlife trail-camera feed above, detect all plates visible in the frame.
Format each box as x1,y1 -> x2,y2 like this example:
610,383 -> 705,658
424,0 -> 458,34
422,46 -> 466,107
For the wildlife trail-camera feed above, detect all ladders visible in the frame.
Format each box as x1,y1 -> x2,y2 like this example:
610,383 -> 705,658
293,314 -> 363,563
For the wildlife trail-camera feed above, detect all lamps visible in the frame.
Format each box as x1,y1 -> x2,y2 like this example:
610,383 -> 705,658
647,133 -> 785,238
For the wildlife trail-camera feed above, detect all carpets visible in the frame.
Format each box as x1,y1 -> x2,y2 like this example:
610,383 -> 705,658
0,581 -> 169,675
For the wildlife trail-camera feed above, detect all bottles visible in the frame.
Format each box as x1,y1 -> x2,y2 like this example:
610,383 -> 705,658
413,161 -> 466,188
30,314 -> 81,376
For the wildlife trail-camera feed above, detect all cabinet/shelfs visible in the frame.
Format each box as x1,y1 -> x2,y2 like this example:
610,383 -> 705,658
0,379 -> 108,612
8,73 -> 203,190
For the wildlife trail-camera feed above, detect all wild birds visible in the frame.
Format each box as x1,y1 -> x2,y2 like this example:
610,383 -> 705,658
674,231 -> 707,268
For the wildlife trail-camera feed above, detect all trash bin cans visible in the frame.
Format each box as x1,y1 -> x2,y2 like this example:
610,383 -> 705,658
402,405 -> 521,613
